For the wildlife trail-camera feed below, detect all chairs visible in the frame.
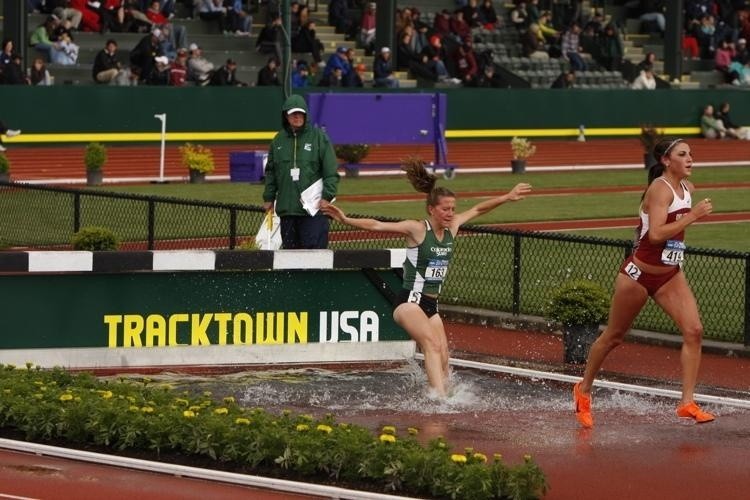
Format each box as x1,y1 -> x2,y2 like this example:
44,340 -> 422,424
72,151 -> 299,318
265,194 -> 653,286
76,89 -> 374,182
395,0 -> 750,89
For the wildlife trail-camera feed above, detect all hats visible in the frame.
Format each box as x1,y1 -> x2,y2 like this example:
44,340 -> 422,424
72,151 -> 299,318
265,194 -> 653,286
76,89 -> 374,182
464,35 -> 473,41
287,107 -> 307,116
11,53 -> 23,58
226,58 -> 236,64
151,28 -> 161,38
188,44 -> 200,51
338,47 -> 348,54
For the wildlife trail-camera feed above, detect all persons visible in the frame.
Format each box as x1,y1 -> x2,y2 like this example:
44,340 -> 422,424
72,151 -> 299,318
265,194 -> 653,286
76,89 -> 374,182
401,0 -> 750,89
701,104 -> 726,140
291,1 -> 400,89
717,102 -> 741,139
320,154 -> 532,395
573,126 -> 716,429
0,1 -> 285,86
262,95 -> 337,250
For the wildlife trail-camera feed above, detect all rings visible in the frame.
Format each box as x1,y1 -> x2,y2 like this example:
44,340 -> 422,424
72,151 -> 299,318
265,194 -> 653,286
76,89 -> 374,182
704,198 -> 709,203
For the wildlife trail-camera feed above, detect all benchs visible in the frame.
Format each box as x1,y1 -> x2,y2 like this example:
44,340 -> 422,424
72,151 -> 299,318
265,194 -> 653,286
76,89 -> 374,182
0,0 -> 418,87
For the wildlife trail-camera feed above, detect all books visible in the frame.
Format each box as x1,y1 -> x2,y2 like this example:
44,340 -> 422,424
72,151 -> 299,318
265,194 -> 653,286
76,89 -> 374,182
298,177 -> 336,217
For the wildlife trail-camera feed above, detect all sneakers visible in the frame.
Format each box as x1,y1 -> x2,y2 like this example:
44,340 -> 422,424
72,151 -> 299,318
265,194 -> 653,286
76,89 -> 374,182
234,31 -> 248,36
223,30 -> 230,35
575,382 -> 595,427
228,31 -> 236,36
677,402 -> 716,423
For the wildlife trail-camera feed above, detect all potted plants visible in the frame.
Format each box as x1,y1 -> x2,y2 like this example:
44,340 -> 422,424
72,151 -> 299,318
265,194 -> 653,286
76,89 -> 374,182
332,144 -> 369,178
548,282 -> 610,363
84,143 -> 106,186
639,124 -> 668,169
511,139 -> 537,173
0,154 -> 9,181
179,142 -> 214,184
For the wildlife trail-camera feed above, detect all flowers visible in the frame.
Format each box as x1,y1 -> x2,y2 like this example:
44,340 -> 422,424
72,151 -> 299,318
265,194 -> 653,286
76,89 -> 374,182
0,355 -> 553,487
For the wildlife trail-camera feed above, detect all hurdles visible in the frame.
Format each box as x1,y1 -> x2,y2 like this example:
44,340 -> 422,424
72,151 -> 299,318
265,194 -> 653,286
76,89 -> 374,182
0,247 -> 416,373
